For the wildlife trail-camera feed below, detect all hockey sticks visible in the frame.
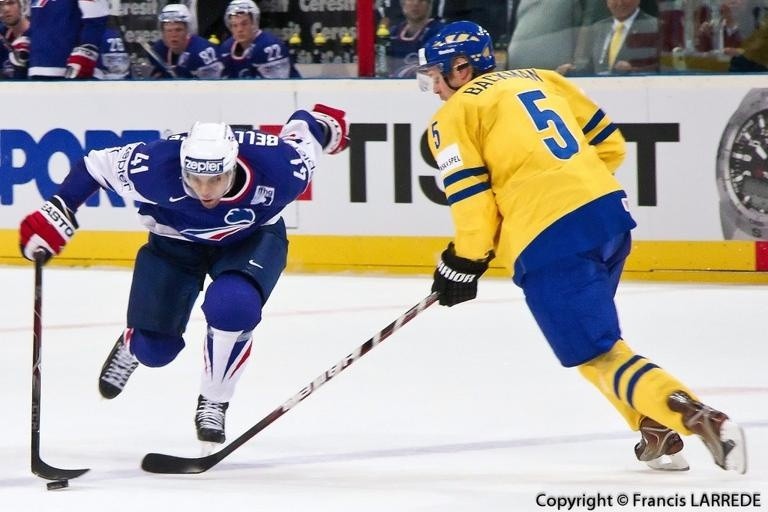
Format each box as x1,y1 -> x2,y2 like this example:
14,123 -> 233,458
137,291 -> 441,475
30,249 -> 89,480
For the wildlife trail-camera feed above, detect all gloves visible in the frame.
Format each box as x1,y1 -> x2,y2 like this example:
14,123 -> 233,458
18,194 -> 81,265
312,102 -> 350,158
11,36 -> 32,64
64,46 -> 100,80
430,250 -> 490,308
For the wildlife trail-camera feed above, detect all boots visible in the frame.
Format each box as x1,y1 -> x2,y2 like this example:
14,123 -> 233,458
189,392 -> 231,458
94,331 -> 142,401
635,415 -> 692,472
666,389 -> 748,476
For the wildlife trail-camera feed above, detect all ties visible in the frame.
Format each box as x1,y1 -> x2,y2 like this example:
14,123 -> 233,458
607,22 -> 627,67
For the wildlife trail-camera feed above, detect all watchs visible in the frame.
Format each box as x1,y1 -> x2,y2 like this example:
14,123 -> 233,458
715,86 -> 768,244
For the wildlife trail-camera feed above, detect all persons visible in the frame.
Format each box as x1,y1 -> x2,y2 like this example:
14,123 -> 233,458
129,4 -> 218,80
216,0 -> 302,79
417,20 -> 749,476
8,0 -> 131,81
18,104 -> 352,443
386,0 -> 445,79
0,0 -> 32,79
683,0 -> 768,72
556,0 -> 665,76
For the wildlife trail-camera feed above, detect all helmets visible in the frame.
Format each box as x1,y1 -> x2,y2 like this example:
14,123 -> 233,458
415,19 -> 499,92
178,119 -> 240,201
156,4 -> 193,45
224,0 -> 261,34
